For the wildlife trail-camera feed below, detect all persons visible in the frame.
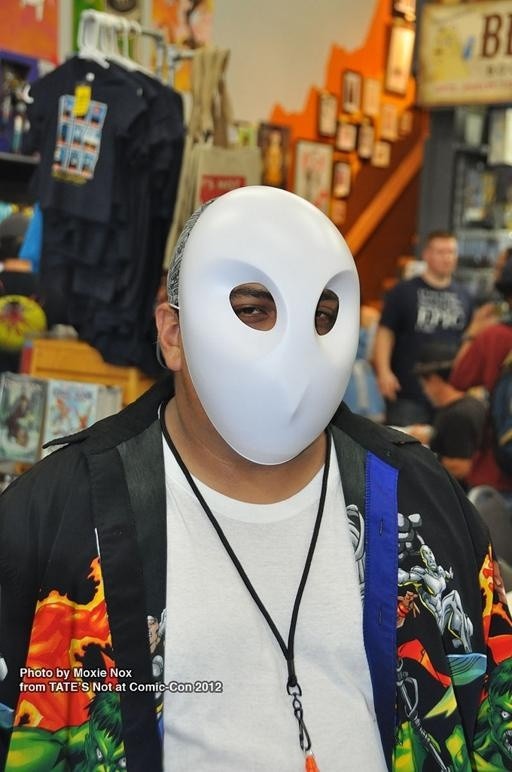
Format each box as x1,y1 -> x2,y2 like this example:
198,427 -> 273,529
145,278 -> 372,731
400,356 -> 489,493
447,246 -> 512,504
0,187 -> 512,772
372,227 -> 478,431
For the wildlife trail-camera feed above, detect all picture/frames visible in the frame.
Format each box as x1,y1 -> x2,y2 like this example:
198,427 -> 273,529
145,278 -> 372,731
254,1 -> 417,235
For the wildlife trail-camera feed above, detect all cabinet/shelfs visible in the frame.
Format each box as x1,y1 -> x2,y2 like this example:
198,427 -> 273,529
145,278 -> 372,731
2,41 -> 43,350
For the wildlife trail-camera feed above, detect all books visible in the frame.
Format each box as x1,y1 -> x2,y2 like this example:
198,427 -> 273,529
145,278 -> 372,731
1,369 -> 124,490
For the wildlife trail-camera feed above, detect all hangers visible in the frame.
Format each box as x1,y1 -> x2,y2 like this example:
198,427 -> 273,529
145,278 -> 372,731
72,2 -> 182,97
